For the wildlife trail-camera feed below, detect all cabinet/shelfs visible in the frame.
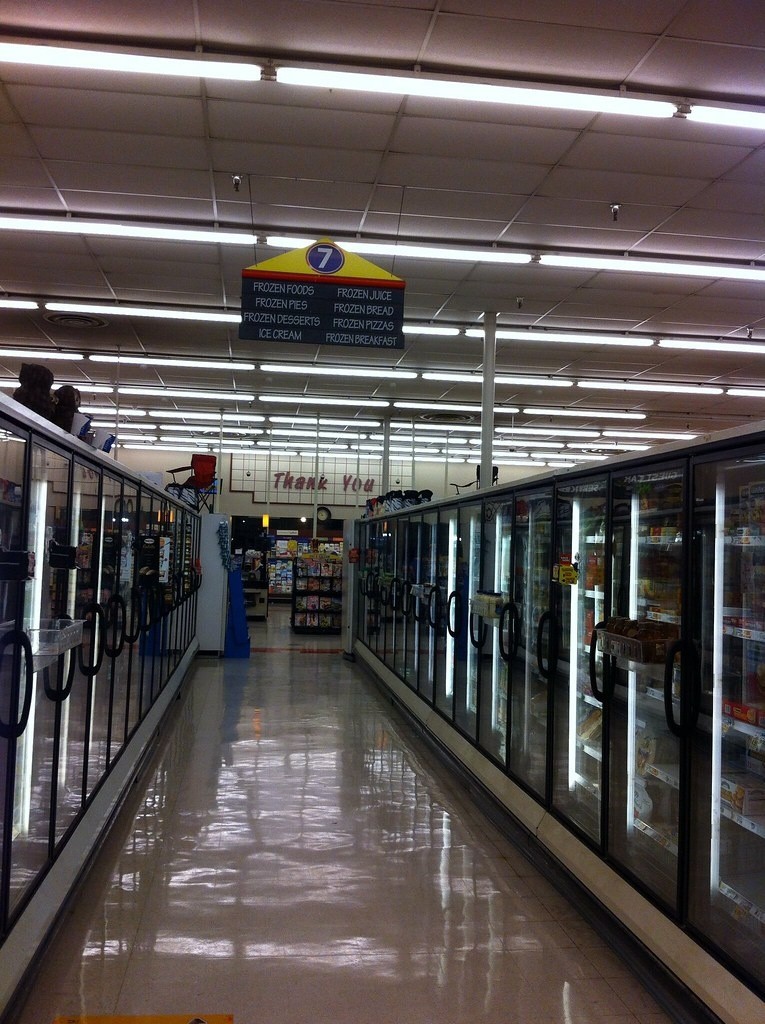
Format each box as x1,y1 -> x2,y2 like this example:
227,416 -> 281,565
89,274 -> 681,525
291,557 -> 341,636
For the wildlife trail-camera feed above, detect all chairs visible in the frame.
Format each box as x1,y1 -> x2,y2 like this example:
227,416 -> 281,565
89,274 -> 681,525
165,453 -> 218,515
450,465 -> 499,495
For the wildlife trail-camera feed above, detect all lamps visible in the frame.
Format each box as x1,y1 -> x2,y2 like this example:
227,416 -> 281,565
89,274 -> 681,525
0,35 -> 764,469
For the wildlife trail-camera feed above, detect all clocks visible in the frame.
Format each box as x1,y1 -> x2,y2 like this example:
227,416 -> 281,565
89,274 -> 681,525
317,507 -> 331,524
